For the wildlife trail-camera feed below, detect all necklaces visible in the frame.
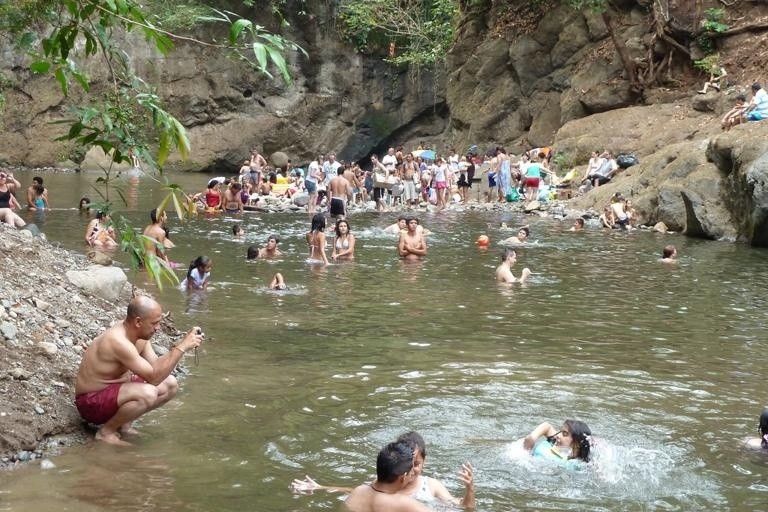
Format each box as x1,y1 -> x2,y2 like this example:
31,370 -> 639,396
370,483 -> 390,494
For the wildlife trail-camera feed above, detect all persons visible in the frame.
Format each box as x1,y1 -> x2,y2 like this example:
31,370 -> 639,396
477,222 -> 531,282
247,234 -> 281,260
720,82 -> 768,133
138,207 -> 180,269
563,190 -> 637,232
1,168 -> 51,228
269,272 -> 286,290
85,207 -> 119,246
735,408 -> 768,466
233,224 -> 244,237
663,245 -> 679,262
74,295 -> 205,447
291,431 -> 475,512
331,220 -> 355,259
336,442 -> 433,512
177,255 -> 212,289
581,149 -> 618,187
181,140 -> 555,222
383,215 -> 431,259
305,213 -> 329,264
523,419 -> 591,474
696,64 -> 729,94
327,214 -> 345,232
76,197 -> 94,210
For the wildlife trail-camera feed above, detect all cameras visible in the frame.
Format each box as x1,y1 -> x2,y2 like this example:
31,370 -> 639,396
1,173 -> 7,177
196,330 -> 205,340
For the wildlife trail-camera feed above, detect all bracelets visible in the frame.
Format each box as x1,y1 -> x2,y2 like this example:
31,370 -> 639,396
175,346 -> 185,354
